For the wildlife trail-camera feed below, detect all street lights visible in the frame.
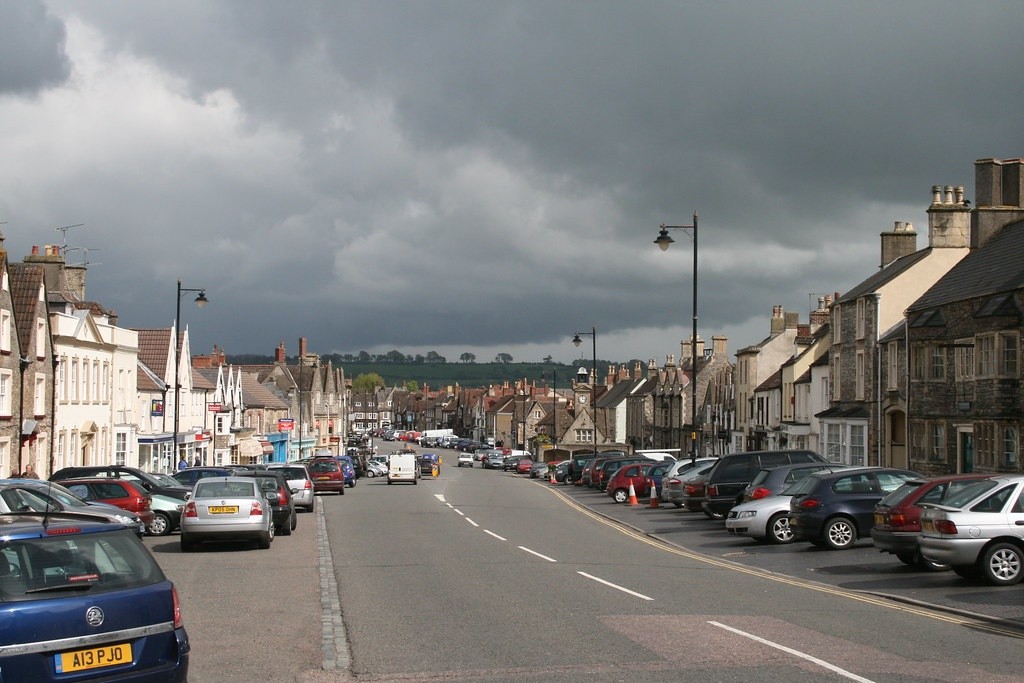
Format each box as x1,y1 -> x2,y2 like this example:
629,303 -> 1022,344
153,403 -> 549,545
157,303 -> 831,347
297,357 -> 319,461
646,210 -> 700,462
554,327 -> 597,459
172,280 -> 209,477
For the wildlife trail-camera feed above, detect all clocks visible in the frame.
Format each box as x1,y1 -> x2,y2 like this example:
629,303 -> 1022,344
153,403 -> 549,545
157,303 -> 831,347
578,395 -> 587,404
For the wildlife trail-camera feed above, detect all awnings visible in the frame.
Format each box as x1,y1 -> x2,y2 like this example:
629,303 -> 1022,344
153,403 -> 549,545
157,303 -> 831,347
260,442 -> 274,453
240,439 -> 263,457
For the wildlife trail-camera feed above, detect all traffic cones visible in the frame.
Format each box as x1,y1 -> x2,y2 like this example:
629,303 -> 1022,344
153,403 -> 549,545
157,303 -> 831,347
625,478 -> 642,506
645,479 -> 664,509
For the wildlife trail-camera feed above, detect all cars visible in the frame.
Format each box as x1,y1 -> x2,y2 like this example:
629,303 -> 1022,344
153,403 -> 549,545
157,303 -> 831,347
683,466 -> 725,520
230,470 -> 297,537
725,466 -> 885,544
1,462 -> 285,543
264,464 -> 317,512
916,475 -> 1024,586
179,476 -> 278,552
529,452 -> 677,502
306,458 -> 347,495
333,455 -> 356,487
347,428 -> 532,485
457,453 -> 476,468
667,461 -> 718,504
870,474 -> 999,573
0,512 -> 191,683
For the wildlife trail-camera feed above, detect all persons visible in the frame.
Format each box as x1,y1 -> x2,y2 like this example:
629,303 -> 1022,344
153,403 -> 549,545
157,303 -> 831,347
8,469 -> 20,477
178,454 -> 189,470
21,464 -> 39,479
194,456 -> 200,466
496,440 -> 504,447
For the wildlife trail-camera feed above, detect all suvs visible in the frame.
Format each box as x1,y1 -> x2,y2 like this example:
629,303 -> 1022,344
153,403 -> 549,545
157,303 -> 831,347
742,463 -> 851,505
701,449 -> 832,514
788,467 -> 930,551
